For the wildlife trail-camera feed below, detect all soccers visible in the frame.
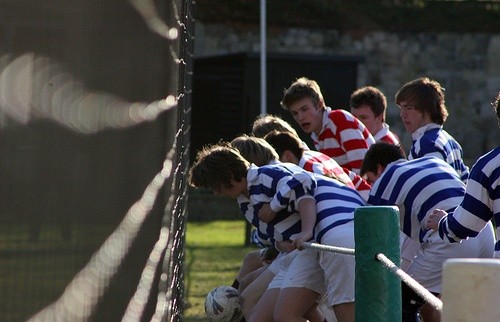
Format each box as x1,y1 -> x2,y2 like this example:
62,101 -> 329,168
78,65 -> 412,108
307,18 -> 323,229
204,285 -> 244,321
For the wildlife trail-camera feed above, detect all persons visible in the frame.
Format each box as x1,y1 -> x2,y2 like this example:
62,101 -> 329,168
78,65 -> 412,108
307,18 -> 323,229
426,92 -> 500,260
188,77 -> 495,322
